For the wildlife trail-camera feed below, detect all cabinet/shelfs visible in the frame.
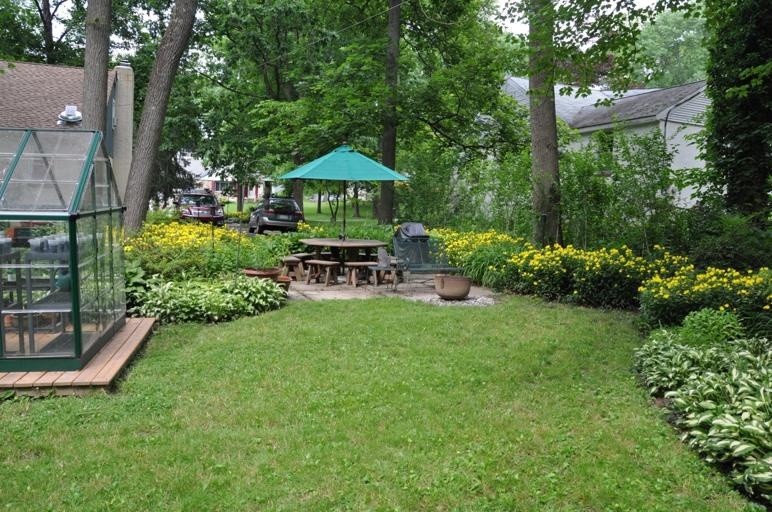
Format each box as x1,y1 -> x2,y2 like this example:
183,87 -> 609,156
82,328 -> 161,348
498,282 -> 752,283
0,248 -> 100,356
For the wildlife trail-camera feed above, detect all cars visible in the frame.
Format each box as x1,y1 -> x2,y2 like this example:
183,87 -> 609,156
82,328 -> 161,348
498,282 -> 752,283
173,188 -> 306,234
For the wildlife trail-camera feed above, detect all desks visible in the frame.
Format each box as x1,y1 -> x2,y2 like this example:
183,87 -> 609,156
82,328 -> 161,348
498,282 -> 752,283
298,239 -> 388,275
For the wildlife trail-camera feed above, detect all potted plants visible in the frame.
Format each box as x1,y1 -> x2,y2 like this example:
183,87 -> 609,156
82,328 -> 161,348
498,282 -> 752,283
241,243 -> 282,281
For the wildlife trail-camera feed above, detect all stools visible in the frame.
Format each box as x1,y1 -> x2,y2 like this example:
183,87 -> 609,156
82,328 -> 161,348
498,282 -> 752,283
280,251 -> 404,288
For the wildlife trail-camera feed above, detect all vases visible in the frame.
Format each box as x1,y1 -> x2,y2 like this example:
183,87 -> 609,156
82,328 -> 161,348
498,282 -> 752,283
0,233 -> 103,253
277,277 -> 291,292
435,275 -> 472,299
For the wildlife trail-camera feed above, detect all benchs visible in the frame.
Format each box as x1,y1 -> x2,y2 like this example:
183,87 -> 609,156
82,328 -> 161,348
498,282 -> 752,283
393,236 -> 464,298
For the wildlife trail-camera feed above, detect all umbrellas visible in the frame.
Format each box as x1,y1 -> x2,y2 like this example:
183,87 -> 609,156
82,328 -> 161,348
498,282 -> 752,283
277,146 -> 411,242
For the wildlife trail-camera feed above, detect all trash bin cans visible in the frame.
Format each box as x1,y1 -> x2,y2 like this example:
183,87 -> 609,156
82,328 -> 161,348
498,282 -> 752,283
393,222 -> 430,272
